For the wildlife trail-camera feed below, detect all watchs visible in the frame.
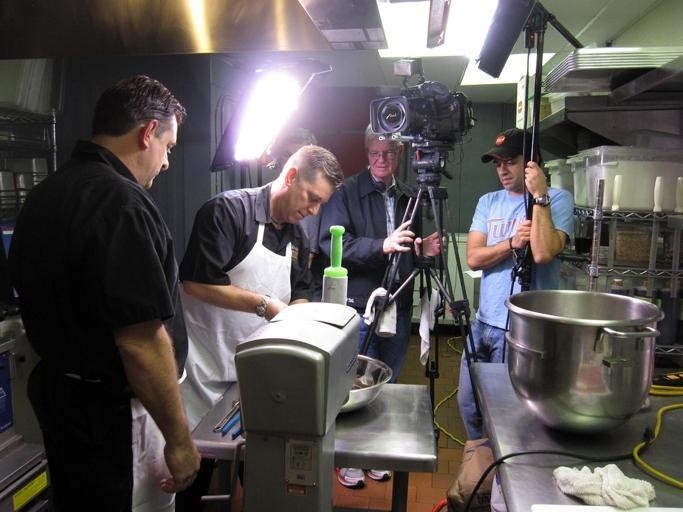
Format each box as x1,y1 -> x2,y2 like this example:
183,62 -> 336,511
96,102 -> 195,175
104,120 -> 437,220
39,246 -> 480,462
254,291 -> 271,321
533,194 -> 552,206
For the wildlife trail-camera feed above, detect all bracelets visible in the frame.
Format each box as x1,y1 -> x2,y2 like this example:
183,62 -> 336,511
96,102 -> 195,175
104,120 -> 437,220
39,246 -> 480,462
508,236 -> 517,252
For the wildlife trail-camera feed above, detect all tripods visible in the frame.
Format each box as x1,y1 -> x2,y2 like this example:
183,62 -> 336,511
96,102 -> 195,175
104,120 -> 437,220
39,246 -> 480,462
359,146 -> 478,448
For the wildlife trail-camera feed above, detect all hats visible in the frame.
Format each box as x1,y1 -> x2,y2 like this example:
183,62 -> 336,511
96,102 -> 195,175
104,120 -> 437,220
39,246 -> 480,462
481,128 -> 539,162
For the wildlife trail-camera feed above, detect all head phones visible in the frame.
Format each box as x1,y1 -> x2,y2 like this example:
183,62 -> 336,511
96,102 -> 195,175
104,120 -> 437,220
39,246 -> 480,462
367,164 -> 396,198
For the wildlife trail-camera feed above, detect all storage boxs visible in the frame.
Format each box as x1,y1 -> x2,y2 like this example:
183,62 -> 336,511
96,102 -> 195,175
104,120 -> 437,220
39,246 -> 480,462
515,76 -> 550,130
543,145 -> 682,209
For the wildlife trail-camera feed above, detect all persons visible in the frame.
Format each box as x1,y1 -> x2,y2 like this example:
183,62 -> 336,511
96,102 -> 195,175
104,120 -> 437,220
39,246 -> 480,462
316,119 -> 448,488
456,126 -> 575,444
273,129 -> 323,271
5,72 -> 200,510
175,144 -> 344,511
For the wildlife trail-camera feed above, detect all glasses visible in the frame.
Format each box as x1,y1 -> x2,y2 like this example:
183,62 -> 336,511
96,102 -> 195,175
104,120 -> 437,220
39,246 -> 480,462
366,146 -> 399,160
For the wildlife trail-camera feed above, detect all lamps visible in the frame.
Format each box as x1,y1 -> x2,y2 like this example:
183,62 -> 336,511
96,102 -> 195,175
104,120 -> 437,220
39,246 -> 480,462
473,1 -> 586,295
211,49 -> 333,191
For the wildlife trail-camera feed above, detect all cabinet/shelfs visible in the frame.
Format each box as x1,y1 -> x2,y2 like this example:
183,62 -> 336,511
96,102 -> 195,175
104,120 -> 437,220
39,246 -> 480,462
554,178 -> 682,356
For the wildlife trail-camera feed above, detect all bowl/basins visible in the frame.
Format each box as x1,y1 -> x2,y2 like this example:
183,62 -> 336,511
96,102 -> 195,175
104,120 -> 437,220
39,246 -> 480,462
339,354 -> 396,413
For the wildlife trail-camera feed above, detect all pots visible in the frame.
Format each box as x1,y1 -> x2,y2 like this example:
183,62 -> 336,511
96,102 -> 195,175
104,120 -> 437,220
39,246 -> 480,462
502,288 -> 667,437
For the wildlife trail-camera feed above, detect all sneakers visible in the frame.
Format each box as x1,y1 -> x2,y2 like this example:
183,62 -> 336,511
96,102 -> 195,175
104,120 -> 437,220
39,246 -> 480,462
335,467 -> 365,489
364,469 -> 392,481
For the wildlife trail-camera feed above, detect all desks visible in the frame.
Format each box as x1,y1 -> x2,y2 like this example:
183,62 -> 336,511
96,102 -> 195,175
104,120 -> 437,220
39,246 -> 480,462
468,361 -> 682,511
190,381 -> 438,512
